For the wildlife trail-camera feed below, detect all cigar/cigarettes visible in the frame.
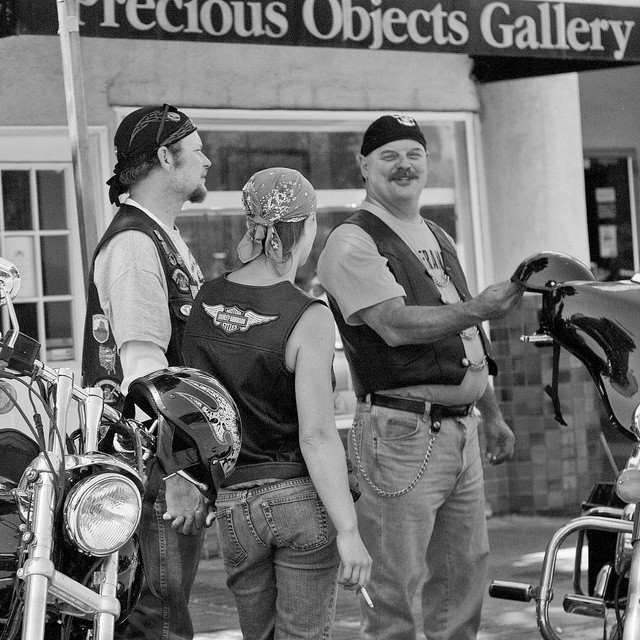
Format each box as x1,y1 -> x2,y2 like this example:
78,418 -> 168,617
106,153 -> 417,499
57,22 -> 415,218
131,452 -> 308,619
360,586 -> 375,608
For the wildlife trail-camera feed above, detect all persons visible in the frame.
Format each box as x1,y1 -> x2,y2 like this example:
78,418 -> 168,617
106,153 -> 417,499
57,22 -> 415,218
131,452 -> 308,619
80,103 -> 212,638
317,115 -> 524,639
163,167 -> 372,640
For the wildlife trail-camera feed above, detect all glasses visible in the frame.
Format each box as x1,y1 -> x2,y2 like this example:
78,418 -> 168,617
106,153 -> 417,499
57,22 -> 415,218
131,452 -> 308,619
156,102 -> 178,146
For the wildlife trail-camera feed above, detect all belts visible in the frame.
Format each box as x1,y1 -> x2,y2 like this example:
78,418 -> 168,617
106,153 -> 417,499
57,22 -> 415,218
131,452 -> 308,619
354,389 -> 475,419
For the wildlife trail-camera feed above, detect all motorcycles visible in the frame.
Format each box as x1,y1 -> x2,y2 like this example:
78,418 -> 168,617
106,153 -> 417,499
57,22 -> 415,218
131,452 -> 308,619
0,256 -> 245,640
488,250 -> 640,640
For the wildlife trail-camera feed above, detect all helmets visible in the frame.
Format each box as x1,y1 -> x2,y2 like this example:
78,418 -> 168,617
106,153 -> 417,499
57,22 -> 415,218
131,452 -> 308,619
510,253 -> 597,297
126,365 -> 244,496
